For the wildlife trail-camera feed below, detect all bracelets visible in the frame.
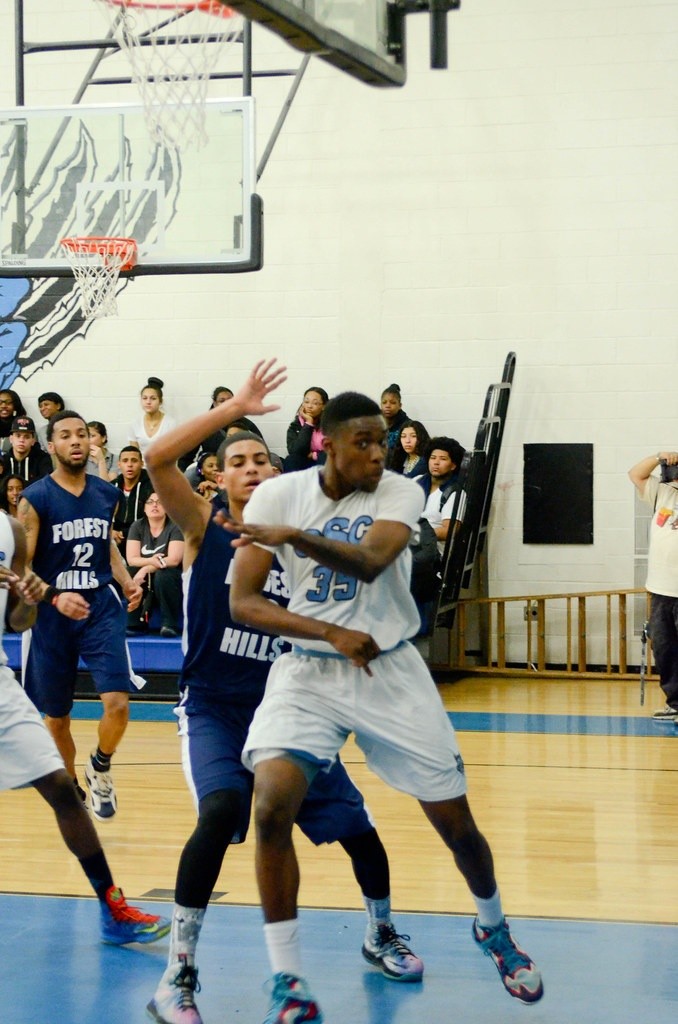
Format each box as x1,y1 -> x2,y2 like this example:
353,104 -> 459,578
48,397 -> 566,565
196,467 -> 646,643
656,453 -> 663,462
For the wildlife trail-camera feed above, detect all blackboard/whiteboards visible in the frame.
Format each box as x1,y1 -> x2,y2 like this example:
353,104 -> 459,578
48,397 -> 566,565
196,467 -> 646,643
523,443 -> 593,544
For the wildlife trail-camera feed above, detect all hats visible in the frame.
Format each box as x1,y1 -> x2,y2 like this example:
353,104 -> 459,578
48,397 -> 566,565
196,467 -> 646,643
10,415 -> 36,433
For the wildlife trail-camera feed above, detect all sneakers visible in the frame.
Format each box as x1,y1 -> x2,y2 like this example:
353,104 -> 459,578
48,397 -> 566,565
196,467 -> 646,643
99,886 -> 170,944
84,757 -> 117,824
146,956 -> 203,1024
360,926 -> 424,982
471,912 -> 544,1005
262,972 -> 322,1024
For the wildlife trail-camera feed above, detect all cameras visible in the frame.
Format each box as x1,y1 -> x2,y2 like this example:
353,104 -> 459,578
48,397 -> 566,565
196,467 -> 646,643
660,459 -> 678,482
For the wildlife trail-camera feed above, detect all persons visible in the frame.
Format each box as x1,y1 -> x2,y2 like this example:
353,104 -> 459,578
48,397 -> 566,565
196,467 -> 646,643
404,436 -> 467,636
627,450 -> 678,728
146,363 -> 423,1024
213,391 -> 545,1024
0,388 -> 67,519
179,382 -> 430,509
81,378 -> 186,638
0,510 -> 171,946
18,410 -> 143,822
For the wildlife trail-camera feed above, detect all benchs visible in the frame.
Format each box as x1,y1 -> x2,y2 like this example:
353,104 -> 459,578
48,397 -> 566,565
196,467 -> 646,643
1,633 -> 185,701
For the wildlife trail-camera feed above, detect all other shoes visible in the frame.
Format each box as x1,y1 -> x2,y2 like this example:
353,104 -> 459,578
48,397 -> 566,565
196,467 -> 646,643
653,705 -> 678,720
126,626 -> 148,637
161,627 -> 180,638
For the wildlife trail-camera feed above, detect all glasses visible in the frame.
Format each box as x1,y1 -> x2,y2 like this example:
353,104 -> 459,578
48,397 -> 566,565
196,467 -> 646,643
145,499 -> 161,505
0,399 -> 12,406
